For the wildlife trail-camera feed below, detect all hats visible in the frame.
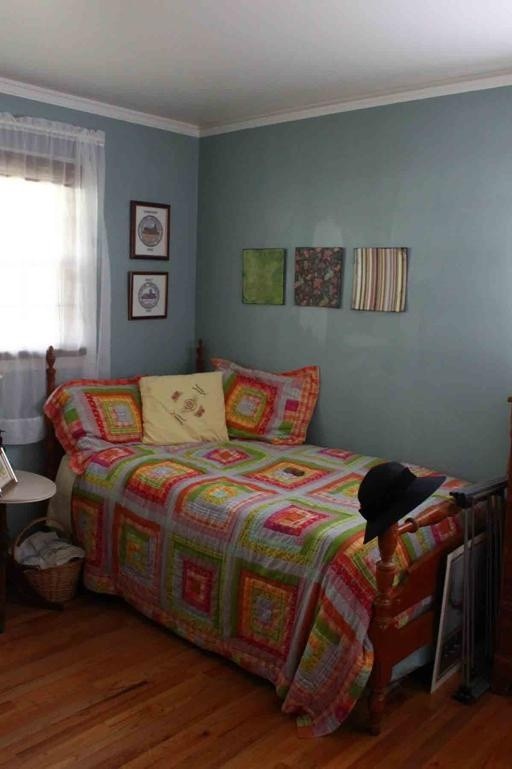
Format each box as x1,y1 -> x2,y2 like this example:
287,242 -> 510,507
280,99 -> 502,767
354,460 -> 444,546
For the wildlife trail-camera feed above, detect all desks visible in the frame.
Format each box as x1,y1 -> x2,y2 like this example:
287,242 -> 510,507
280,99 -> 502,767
0,470 -> 57,633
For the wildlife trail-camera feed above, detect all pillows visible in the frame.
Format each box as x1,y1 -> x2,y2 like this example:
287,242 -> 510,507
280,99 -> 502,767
137,373 -> 231,445
210,358 -> 320,446
44,377 -> 142,473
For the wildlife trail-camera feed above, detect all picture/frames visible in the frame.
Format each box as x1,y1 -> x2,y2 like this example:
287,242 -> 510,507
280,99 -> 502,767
130,200 -> 170,260
128,271 -> 168,320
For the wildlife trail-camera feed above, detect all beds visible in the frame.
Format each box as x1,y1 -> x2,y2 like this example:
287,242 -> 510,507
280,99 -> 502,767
42,337 -> 507,736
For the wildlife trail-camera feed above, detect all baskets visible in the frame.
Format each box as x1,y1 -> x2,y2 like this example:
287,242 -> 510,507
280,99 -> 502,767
6,519 -> 86,608
